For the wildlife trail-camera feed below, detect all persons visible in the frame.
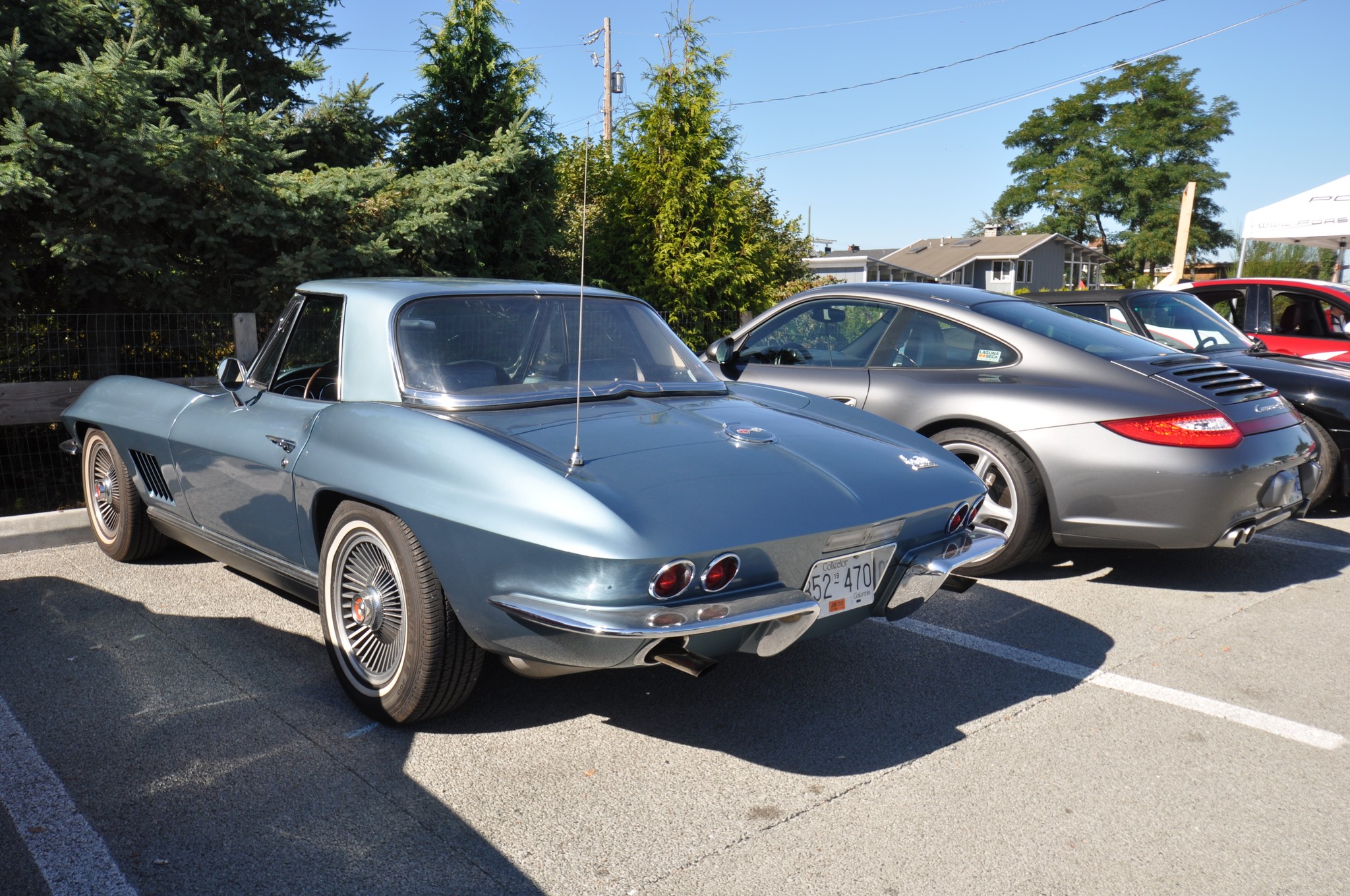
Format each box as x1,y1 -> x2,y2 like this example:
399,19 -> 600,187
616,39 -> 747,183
1277,293 -> 1349,335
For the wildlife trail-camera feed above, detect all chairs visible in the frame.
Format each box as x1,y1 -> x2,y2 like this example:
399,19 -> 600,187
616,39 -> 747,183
901,316 -> 947,373
1277,304 -> 1302,336
559,356 -> 642,383
440,360 -> 513,390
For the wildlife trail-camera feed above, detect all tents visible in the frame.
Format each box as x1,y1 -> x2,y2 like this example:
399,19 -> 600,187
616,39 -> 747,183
1228,174 -> 1350,325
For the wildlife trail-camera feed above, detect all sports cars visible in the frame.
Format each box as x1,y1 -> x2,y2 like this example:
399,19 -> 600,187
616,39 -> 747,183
58,278 -> 991,731
943,277 -> 1350,516
695,282 -> 1325,578
